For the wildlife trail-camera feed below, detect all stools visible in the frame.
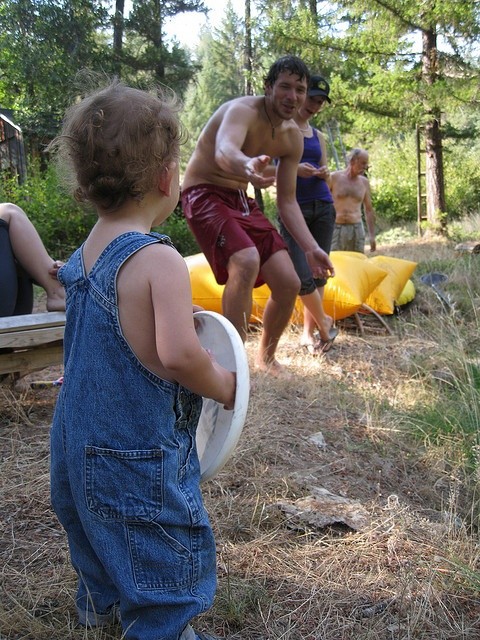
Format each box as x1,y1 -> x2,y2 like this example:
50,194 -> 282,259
1,310 -> 70,381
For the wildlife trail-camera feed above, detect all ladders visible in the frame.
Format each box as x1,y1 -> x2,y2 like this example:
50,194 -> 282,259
325,118 -> 349,170
413,122 -> 428,236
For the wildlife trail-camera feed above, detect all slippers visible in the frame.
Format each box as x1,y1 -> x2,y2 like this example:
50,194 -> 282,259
319,328 -> 338,352
298,343 -> 319,356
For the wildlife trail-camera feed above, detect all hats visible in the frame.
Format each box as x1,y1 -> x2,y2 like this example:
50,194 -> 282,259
307,76 -> 331,103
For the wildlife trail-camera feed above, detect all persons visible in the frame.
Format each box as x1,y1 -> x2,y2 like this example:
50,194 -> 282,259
0,203 -> 66,317
274,76 -> 339,357
45,85 -> 237,640
322,148 -> 376,253
180,56 -> 335,382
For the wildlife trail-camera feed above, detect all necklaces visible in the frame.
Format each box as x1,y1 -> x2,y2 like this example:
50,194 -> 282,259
264,97 -> 284,140
297,119 -> 310,131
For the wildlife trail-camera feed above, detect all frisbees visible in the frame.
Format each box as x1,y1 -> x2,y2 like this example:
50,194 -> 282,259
186,307 -> 251,483
